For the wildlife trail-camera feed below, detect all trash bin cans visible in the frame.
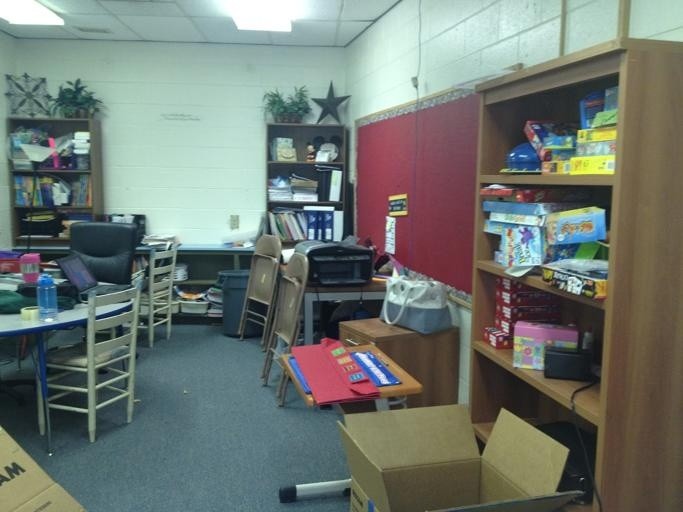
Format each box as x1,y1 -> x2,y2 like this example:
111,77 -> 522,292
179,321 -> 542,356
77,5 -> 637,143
218,270 -> 260,337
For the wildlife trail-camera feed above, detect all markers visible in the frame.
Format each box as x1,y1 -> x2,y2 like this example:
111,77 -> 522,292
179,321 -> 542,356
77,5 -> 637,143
278,480 -> 351,503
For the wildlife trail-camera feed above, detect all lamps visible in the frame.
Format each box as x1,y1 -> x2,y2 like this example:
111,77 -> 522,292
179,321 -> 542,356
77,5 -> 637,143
16,140 -> 56,258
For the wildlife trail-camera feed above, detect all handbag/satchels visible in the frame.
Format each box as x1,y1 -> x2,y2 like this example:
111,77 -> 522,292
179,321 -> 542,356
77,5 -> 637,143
379,277 -> 452,335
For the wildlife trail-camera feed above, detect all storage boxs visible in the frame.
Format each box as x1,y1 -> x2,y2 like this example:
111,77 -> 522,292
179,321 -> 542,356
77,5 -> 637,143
500,88 -> 620,175
478,181 -> 613,299
330,398 -> 586,510
0,420 -> 85,512
479,278 -> 605,372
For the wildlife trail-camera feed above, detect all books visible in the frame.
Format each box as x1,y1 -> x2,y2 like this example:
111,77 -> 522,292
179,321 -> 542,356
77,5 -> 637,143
269,134 -> 344,245
14,175 -> 92,241
130,234 -> 224,319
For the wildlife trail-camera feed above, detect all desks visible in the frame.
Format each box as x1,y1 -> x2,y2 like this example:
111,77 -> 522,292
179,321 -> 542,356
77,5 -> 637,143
272,344 -> 426,505
0,268 -> 135,435
299,279 -> 389,354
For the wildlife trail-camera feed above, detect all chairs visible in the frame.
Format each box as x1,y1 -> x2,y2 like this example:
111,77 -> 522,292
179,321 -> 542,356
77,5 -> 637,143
120,249 -> 180,348
66,222 -> 141,297
256,252 -> 310,408
234,229 -> 284,351
29,281 -> 144,443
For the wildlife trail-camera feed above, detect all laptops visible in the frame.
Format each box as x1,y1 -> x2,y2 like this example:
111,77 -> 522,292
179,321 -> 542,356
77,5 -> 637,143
55,254 -> 134,301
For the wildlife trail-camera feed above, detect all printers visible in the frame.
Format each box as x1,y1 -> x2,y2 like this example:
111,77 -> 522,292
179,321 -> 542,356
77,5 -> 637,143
294,240 -> 373,287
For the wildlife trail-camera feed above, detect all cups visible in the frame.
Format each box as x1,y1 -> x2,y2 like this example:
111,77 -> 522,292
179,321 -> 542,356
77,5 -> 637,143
19,252 -> 40,283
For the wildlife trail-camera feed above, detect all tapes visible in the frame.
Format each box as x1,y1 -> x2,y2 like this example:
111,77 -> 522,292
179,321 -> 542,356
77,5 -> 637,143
20,306 -> 37,319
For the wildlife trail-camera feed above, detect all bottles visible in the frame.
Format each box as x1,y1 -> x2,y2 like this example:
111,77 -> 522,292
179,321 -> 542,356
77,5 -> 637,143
34,272 -> 57,322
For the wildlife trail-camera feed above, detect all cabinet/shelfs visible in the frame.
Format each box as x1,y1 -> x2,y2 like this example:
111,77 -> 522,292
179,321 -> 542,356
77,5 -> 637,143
261,122 -> 350,247
336,316 -> 462,409
135,242 -> 263,329
4,112 -> 106,249
462,35 -> 682,511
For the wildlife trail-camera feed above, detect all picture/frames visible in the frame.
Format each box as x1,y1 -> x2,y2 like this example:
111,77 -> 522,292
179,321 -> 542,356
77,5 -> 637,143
58,254 -> 96,293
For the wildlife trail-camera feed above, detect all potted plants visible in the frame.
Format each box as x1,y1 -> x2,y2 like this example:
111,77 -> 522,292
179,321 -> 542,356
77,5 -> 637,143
261,86 -> 313,122
48,83 -> 104,118
